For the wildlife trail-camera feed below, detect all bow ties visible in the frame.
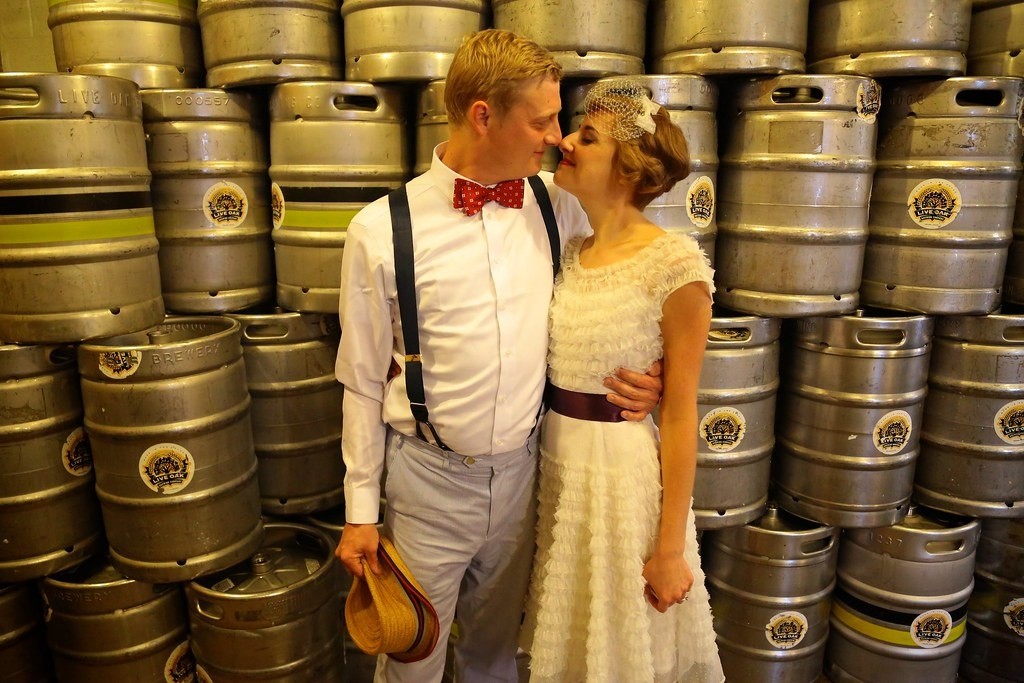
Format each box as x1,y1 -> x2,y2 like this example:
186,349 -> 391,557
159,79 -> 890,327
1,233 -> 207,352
453,178 -> 524,217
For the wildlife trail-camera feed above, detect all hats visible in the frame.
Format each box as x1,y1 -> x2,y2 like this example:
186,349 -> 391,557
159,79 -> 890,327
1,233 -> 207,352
344,534 -> 440,663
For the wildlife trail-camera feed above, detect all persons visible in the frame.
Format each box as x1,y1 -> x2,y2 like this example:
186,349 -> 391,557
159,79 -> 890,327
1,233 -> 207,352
334,28 -> 662,683
515,78 -> 726,683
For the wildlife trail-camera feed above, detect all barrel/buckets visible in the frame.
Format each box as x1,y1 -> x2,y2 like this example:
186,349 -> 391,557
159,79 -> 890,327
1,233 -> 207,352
0,0 -> 1024,683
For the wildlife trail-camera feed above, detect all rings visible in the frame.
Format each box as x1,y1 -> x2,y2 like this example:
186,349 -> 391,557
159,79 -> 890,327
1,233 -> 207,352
681,596 -> 688,601
